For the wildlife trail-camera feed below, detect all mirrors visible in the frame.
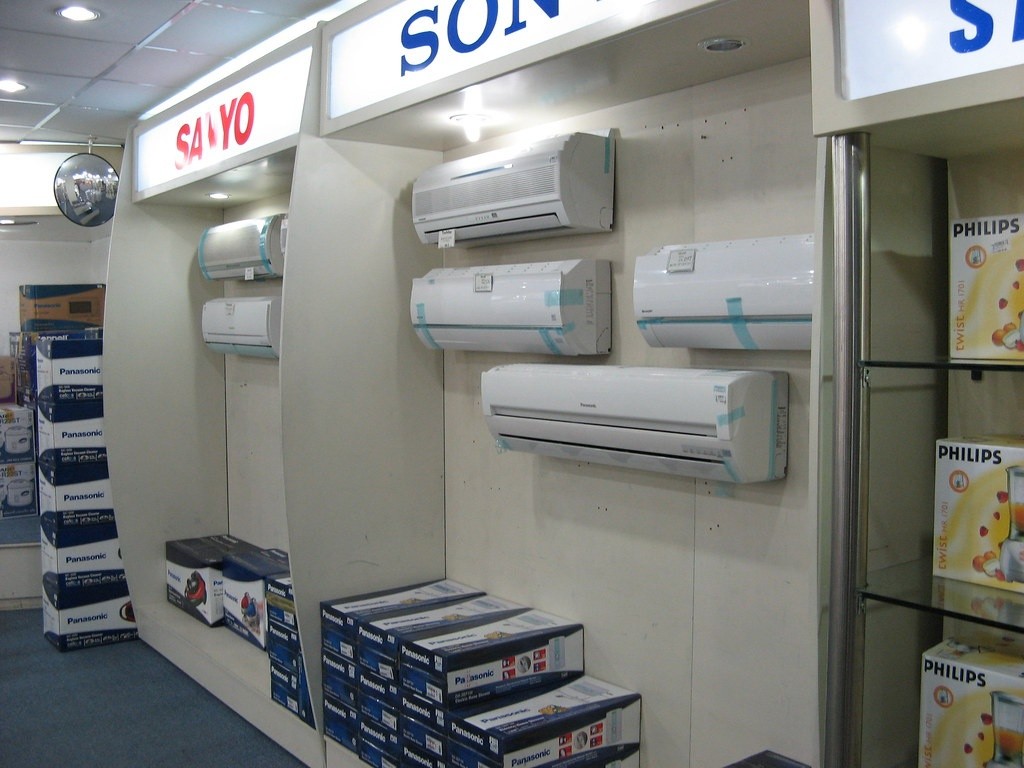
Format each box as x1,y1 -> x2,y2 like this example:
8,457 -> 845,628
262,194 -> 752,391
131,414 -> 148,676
54,152 -> 119,227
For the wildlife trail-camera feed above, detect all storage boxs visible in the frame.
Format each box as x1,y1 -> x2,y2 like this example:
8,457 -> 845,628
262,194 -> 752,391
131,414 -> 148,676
167,533 -> 315,733
320,576 -> 647,768
918,638 -> 1023,768
933,435 -> 1023,594
947,215 -> 1024,365
0,283 -> 137,652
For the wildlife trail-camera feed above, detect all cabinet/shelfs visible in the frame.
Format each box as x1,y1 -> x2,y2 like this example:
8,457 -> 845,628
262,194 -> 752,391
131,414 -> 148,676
851,97 -> 1023,768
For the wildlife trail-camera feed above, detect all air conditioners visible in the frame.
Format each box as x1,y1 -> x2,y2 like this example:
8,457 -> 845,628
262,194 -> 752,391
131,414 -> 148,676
202,297 -> 280,357
480,363 -> 789,485
199,209 -> 285,282
630,239 -> 815,351
412,126 -> 619,245
408,258 -> 612,356
